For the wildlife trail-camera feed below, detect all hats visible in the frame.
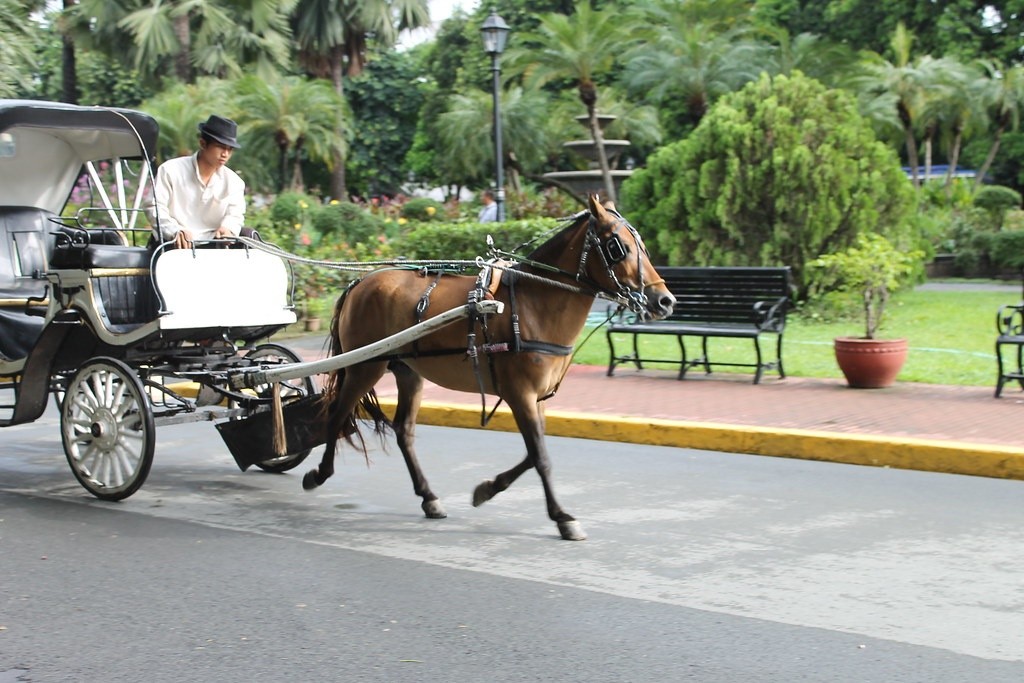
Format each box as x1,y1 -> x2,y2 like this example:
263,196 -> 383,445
197,114 -> 243,151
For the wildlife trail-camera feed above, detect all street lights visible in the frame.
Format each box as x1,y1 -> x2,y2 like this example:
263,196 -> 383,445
480,5 -> 508,224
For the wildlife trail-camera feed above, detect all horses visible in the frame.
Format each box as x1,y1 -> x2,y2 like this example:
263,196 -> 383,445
304,195 -> 676,542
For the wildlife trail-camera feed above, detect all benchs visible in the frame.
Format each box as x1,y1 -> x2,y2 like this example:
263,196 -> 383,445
48,208 -> 152,277
994,304 -> 1024,399
607,266 -> 794,384
0,206 -> 129,305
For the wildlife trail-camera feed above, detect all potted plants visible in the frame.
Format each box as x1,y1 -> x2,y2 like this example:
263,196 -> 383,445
805,231 -> 925,388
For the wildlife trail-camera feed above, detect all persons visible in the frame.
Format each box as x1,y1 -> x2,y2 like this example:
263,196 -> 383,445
142,115 -> 246,250
476,191 -> 498,224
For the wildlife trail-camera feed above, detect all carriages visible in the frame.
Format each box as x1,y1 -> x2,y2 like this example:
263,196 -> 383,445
0,98 -> 675,554
